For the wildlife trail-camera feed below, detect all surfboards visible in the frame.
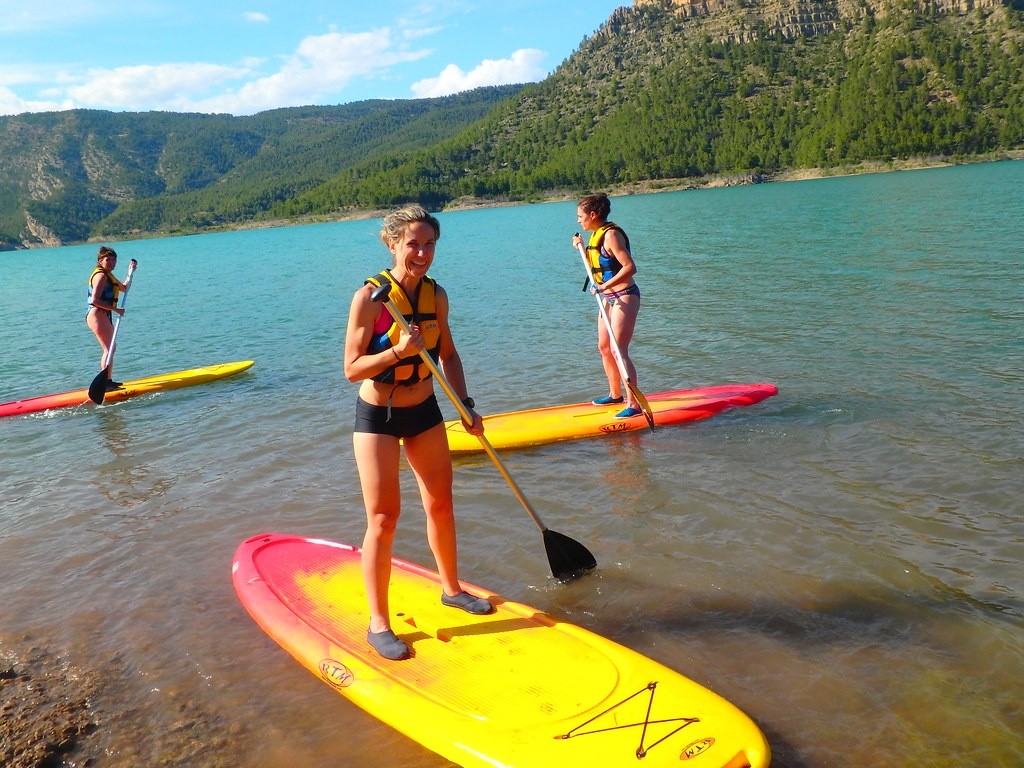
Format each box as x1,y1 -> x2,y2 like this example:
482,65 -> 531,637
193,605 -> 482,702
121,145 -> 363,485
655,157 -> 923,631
398,379 -> 780,451
232,535 -> 772,768
2,360 -> 260,418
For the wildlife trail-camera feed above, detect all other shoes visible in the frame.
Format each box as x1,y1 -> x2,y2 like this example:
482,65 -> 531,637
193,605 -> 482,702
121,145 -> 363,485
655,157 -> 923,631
368,630 -> 409,659
592,395 -> 624,405
616,405 -> 643,419
104,378 -> 123,391
440,591 -> 493,615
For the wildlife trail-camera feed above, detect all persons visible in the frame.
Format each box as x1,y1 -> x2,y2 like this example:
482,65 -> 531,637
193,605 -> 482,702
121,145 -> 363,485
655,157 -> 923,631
573,193 -> 643,418
86,246 -> 136,391
344,204 -> 492,659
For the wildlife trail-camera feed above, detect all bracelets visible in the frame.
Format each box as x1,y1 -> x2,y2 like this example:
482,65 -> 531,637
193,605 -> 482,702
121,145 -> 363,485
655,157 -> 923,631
461,397 -> 475,408
391,347 -> 402,361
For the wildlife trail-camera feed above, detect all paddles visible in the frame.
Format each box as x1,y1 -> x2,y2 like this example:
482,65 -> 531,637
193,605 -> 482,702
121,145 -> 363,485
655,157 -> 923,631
87,259 -> 141,404
574,232 -> 656,432
373,281 -> 595,581
